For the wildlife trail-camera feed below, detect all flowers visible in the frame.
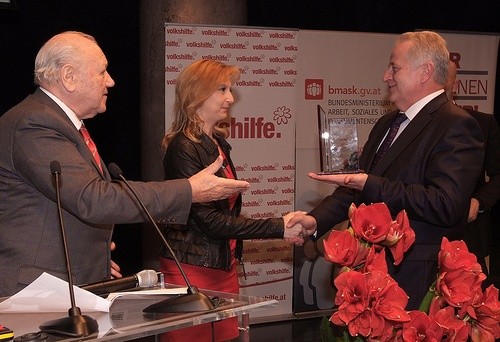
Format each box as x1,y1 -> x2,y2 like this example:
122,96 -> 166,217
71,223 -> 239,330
320,203 -> 500,342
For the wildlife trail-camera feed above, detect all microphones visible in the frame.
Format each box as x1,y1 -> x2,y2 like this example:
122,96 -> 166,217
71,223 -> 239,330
107,163 -> 215,314
38,160 -> 99,337
79,269 -> 158,295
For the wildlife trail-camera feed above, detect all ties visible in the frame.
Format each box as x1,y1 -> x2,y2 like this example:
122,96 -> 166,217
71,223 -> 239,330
80,124 -> 104,179
371,112 -> 407,174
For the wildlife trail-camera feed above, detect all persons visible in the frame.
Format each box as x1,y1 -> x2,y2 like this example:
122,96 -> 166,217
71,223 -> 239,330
162,59 -> 307,342
0,31 -> 251,298
445,59 -> 500,292
285,31 -> 484,315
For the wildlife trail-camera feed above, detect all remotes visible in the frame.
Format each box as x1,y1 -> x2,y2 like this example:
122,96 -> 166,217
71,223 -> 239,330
0,325 -> 15,342
